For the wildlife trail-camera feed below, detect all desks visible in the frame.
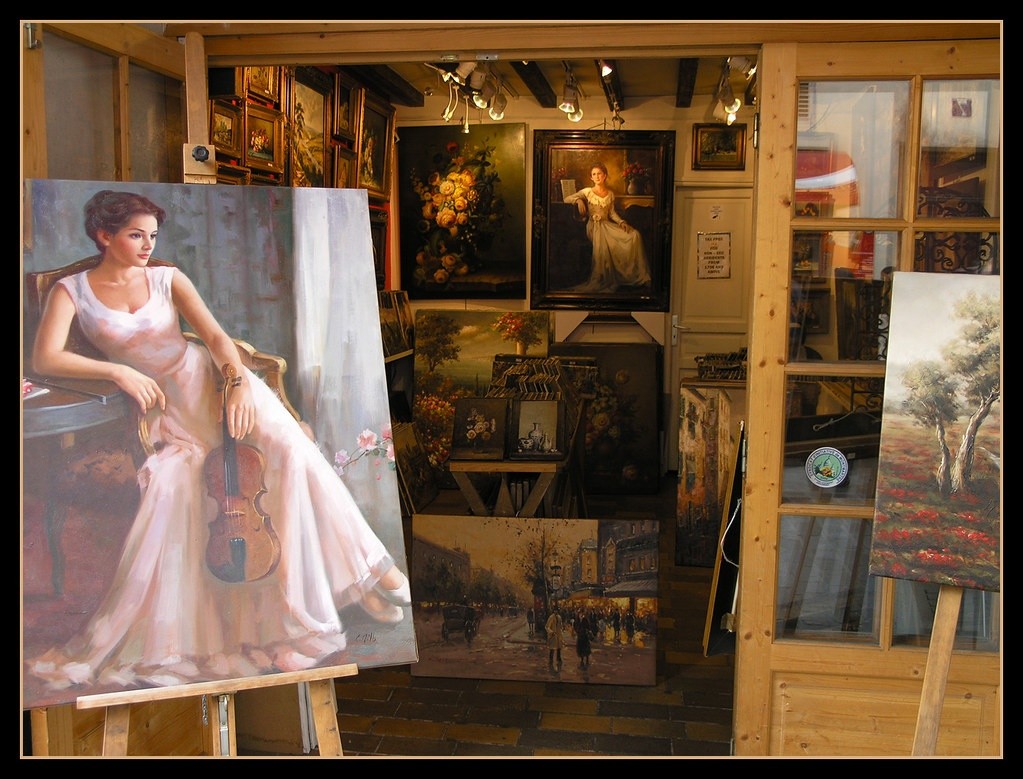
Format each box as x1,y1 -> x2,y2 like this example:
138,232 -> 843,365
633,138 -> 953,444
451,399 -> 586,518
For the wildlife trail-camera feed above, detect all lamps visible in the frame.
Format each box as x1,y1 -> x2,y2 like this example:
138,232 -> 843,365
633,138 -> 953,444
720,55 -> 758,127
600,59 -> 612,77
560,70 -> 584,122
425,62 -> 507,134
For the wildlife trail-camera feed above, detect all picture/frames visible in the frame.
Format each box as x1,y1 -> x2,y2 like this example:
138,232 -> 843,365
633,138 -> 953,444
928,79 -> 989,179
206,65 -> 396,289
835,267 -> 885,360
788,144 -> 832,337
530,129 -> 677,311
691,123 -> 747,171
